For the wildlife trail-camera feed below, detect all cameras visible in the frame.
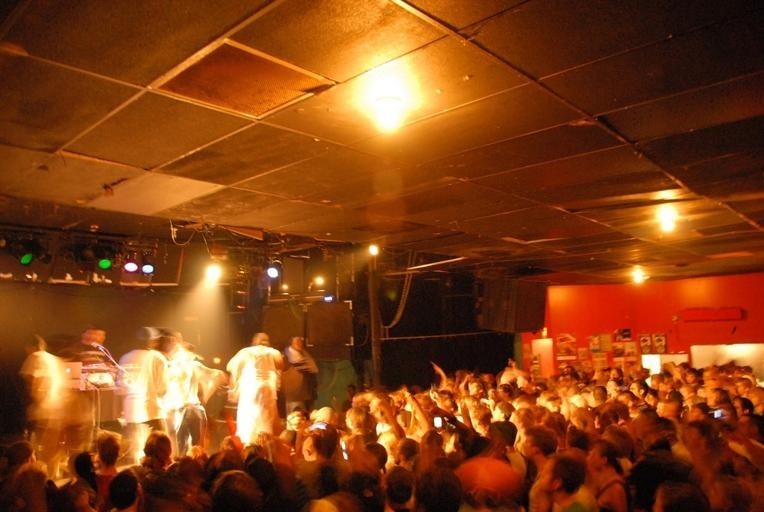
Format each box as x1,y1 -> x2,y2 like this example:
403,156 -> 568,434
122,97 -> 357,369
713,409 -> 722,419
431,414 -> 446,430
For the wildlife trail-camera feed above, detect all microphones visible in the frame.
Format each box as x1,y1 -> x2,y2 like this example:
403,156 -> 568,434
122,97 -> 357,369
90,342 -> 103,350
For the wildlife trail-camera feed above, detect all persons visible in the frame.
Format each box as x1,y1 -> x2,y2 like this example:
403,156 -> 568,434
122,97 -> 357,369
19,329 -> 319,473
0,360 -> 764,512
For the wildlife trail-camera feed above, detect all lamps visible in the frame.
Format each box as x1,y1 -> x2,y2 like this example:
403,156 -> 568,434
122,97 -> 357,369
0,223 -> 156,275
267,256 -> 284,279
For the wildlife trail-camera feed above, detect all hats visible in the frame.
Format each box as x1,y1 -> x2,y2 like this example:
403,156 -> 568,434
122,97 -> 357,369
135,326 -> 159,340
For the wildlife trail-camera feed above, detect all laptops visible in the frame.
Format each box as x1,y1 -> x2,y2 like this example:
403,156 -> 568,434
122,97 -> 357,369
65,361 -> 82,380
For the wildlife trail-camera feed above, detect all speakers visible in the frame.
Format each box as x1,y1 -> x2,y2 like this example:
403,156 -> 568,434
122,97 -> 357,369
481,277 -> 548,335
